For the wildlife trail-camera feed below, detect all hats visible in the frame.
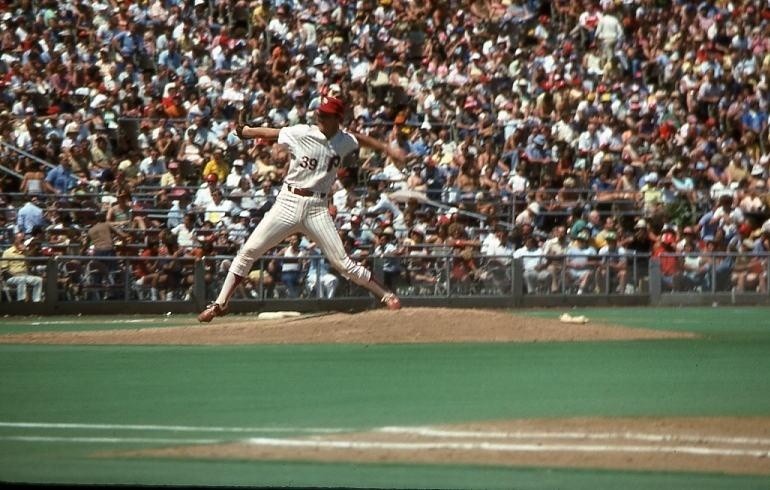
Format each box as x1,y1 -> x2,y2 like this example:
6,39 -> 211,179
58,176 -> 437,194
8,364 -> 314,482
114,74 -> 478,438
0,0 -> 770,291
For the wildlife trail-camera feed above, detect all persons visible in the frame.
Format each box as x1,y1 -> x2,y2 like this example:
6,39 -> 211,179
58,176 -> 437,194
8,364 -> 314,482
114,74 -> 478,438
196,94 -> 408,325
0,1 -> 770,302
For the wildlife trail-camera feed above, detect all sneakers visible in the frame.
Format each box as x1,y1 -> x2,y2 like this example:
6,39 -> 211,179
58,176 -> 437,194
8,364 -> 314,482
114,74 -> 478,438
197,299 -> 230,324
380,292 -> 402,312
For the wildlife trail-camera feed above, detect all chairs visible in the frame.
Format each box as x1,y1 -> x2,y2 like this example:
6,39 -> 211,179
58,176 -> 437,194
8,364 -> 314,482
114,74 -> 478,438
0,0 -> 770,292
